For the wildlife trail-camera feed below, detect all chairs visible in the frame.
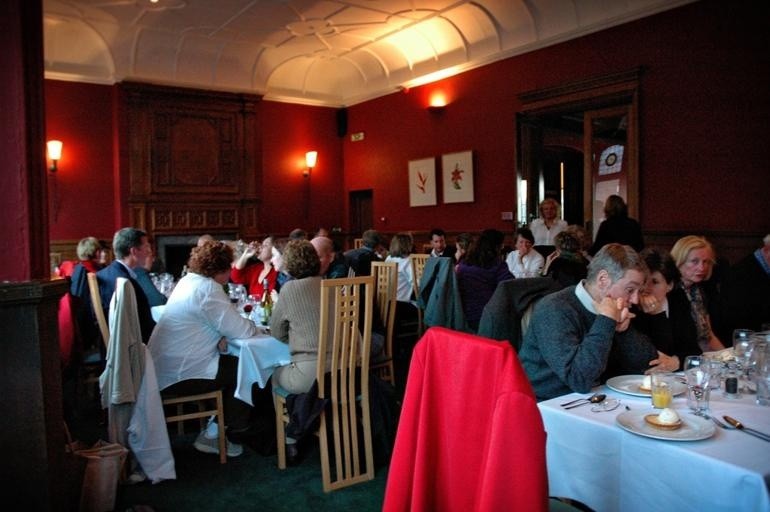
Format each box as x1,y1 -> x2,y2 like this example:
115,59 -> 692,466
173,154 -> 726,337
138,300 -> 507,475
384,326 -> 576,512
423,245 -> 432,252
370,262 -> 397,391
275,276 -> 375,492
354,239 -> 363,249
100,276 -> 225,464
87,272 -> 185,437
410,254 -> 476,338
534,245 -> 555,259
54,260 -> 75,356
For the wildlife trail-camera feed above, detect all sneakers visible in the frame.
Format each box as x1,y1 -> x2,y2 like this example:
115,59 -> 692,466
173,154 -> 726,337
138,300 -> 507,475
193,431 -> 243,457
277,442 -> 299,469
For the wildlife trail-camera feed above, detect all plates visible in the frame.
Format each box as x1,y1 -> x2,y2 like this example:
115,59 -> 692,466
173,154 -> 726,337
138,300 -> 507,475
605,373 -> 688,398
615,406 -> 718,443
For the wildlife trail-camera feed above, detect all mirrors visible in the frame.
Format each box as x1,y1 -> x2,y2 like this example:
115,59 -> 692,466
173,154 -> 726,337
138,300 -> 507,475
585,104 -> 640,242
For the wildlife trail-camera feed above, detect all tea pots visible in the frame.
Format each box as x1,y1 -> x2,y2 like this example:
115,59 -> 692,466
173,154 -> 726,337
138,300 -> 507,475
225,282 -> 248,310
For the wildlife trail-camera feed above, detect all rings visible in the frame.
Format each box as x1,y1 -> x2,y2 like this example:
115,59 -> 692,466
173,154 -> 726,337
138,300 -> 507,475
650,303 -> 657,307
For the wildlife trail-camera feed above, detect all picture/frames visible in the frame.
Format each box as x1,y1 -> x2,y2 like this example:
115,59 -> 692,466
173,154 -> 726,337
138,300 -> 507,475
441,150 -> 475,204
350,131 -> 365,141
408,157 -> 437,207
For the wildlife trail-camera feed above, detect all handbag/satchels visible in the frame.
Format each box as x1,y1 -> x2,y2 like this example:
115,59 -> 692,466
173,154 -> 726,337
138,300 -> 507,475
66,424 -> 128,512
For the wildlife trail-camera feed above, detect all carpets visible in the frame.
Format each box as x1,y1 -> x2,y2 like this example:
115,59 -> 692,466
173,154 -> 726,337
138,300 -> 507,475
115,429 -> 389,511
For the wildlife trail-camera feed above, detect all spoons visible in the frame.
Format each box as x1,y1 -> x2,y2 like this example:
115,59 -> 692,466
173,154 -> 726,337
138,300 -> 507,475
564,392 -> 607,411
723,414 -> 770,439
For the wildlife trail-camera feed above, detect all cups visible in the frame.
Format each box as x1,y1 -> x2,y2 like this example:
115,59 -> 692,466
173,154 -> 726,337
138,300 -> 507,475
236,238 -> 262,256
650,369 -> 675,409
699,353 -> 724,391
732,327 -> 754,365
683,355 -> 712,420
240,294 -> 256,322
753,360 -> 770,409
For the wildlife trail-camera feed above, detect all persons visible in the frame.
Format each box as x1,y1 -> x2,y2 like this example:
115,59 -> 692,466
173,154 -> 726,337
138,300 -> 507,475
343,229 -> 388,276
270,242 -> 295,304
506,230 -> 544,278
545,232 -> 590,285
454,235 -> 476,271
518,244 -> 659,404
262,238 -> 364,465
135,245 -> 169,306
149,241 -> 257,456
543,225 -> 593,274
595,195 -> 645,255
95,227 -> 159,365
429,229 -> 455,258
71,236 -> 103,349
670,236 -> 725,352
386,231 -> 426,319
723,236 -> 770,345
454,231 -> 514,331
233,235 -> 280,299
529,198 -> 566,245
309,237 -> 384,359
630,248 -> 704,374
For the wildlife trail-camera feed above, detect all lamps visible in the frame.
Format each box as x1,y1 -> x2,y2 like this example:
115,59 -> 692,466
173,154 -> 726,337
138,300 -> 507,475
303,151 -> 318,176
46,140 -> 62,171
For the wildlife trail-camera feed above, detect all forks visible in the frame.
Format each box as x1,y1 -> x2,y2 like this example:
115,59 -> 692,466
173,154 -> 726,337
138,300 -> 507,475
710,415 -> 768,444
560,391 -> 598,408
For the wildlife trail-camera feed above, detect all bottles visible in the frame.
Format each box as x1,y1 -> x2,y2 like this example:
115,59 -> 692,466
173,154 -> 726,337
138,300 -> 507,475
260,279 -> 274,325
